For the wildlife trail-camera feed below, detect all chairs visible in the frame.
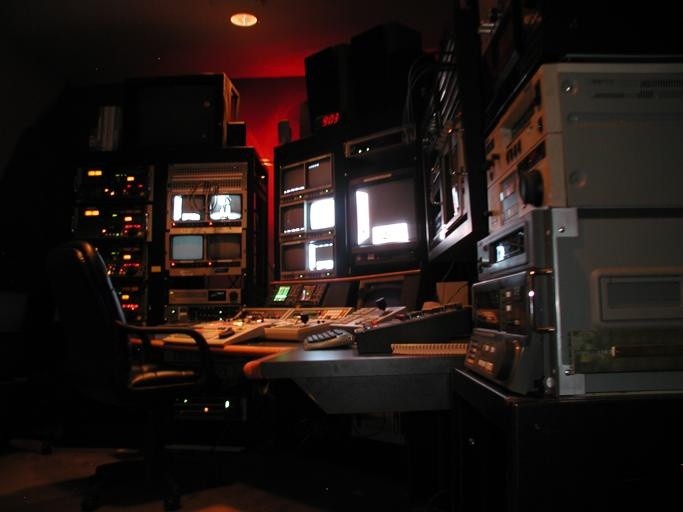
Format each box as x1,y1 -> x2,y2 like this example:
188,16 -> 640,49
62,242 -> 234,508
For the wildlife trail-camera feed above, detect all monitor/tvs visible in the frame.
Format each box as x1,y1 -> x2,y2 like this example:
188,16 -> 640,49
164,154 -> 345,278
347,166 -> 420,256
